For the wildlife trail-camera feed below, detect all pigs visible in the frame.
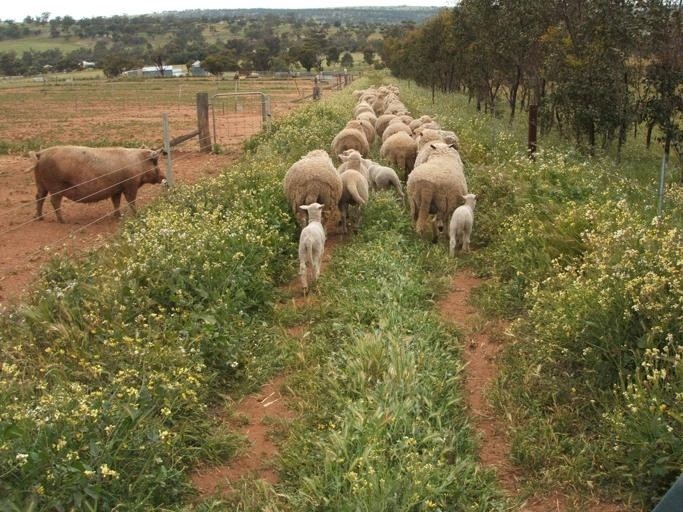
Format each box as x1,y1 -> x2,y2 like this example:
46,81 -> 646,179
23,145 -> 169,223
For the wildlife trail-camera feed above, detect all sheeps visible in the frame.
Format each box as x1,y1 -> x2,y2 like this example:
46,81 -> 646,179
295,202 -> 328,288
280,82 -> 478,258
